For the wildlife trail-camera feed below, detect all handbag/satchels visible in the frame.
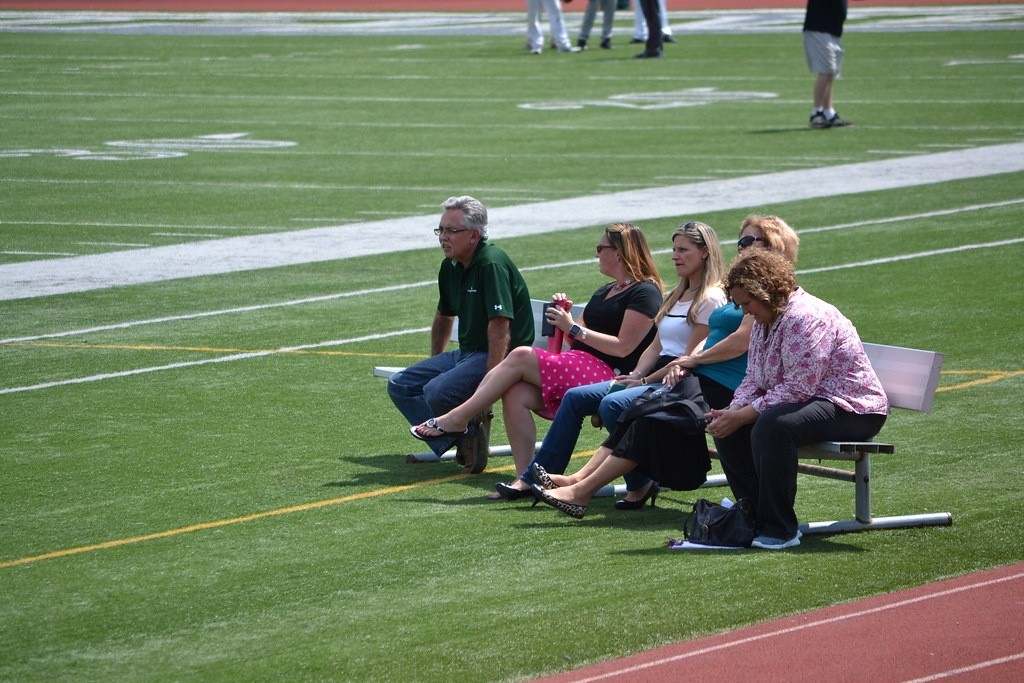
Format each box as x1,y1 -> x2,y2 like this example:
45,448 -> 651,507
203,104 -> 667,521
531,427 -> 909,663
683,497 -> 754,546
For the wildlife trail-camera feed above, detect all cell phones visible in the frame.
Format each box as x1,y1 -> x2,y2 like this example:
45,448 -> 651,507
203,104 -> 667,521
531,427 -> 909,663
541,303 -> 557,336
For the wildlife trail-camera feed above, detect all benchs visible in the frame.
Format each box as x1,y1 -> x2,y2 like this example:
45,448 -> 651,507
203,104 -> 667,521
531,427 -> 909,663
372,298 -> 955,537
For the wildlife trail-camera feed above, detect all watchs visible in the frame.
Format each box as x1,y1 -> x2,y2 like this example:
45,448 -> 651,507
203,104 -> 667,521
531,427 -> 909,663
568,324 -> 581,339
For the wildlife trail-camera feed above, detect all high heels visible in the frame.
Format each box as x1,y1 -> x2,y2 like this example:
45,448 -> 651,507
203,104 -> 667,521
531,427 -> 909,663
495,481 -> 541,509
614,481 -> 660,509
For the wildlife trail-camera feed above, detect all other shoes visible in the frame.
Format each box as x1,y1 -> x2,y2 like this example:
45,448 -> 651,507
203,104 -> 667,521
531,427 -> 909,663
636,50 -> 655,57
809,111 -> 828,128
562,46 -> 581,53
828,114 -> 847,125
663,36 -> 674,42
578,41 -> 587,48
530,47 -> 541,54
533,462 -> 559,490
456,423 -> 489,474
631,38 -> 642,43
531,483 -> 587,519
602,41 -> 612,49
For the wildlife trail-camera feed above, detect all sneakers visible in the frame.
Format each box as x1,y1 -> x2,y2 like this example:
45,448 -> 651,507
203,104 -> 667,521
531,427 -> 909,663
751,536 -> 801,550
794,529 -> 803,538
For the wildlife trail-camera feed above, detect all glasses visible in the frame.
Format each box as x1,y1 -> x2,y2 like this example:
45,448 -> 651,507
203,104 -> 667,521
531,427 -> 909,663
433,228 -> 470,236
596,245 -> 614,253
738,236 -> 767,248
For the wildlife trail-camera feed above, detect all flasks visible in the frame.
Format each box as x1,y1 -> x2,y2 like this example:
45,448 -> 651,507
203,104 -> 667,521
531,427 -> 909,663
547,299 -> 567,354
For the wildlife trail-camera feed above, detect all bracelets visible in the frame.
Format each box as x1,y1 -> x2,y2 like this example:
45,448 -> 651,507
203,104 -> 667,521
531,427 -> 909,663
634,370 -> 643,377
641,377 -> 648,385
581,329 -> 586,343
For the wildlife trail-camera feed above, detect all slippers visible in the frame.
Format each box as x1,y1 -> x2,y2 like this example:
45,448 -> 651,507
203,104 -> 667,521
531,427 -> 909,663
409,417 -> 469,441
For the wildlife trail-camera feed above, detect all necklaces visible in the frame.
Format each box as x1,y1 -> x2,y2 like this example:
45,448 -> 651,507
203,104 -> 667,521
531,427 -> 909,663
613,278 -> 635,291
688,286 -> 700,293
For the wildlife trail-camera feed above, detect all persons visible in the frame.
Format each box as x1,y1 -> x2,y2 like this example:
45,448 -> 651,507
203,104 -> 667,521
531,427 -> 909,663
803,0 -> 848,128
409,223 -> 663,499
704,248 -> 888,548
577,0 -> 618,50
532,215 -> 800,519
495,221 -> 727,510
525,0 -> 581,54
630,0 -> 676,58
387,196 -> 536,474
